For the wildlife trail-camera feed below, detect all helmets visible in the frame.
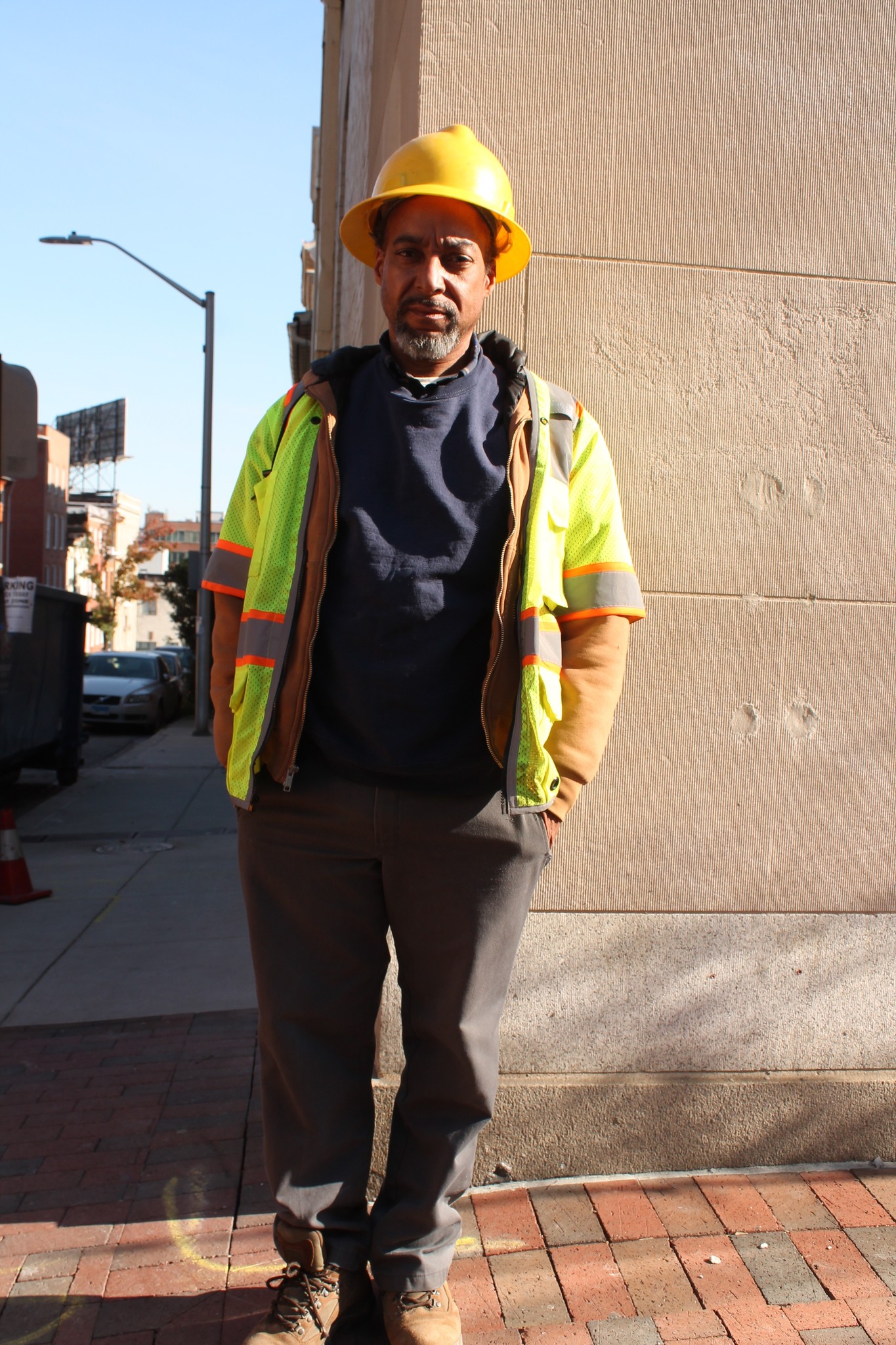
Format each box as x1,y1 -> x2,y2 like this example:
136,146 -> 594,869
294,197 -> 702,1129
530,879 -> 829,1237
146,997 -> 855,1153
339,124 -> 531,284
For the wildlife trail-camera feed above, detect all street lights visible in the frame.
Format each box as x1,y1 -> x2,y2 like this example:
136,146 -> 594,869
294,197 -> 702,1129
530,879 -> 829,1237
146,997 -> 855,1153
37,230 -> 212,735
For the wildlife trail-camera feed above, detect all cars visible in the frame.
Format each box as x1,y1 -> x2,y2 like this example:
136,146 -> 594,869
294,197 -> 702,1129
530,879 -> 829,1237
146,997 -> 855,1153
150,646 -> 192,716
81,651 -> 182,735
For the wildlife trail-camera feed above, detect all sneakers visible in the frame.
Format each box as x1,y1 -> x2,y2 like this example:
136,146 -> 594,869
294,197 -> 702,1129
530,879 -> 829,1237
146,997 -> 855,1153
243,1221 -> 370,1345
379,1280 -> 464,1345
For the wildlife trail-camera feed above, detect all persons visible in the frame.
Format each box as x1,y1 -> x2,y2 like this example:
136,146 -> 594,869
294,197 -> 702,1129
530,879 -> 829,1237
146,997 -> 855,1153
201,124 -> 646,1345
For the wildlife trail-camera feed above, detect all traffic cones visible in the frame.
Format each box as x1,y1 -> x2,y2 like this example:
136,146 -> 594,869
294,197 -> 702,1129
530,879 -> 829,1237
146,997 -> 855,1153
0,802 -> 55,906
